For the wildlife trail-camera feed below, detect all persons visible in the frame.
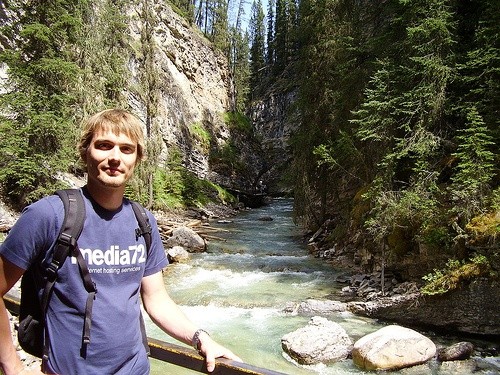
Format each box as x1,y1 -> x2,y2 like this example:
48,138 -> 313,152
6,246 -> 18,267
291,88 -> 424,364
0,109 -> 243,375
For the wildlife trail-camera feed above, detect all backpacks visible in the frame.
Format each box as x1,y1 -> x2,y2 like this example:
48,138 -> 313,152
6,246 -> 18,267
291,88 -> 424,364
17,189 -> 153,360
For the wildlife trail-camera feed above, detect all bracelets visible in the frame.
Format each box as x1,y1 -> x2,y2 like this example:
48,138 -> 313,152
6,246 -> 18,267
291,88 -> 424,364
191,329 -> 210,350
